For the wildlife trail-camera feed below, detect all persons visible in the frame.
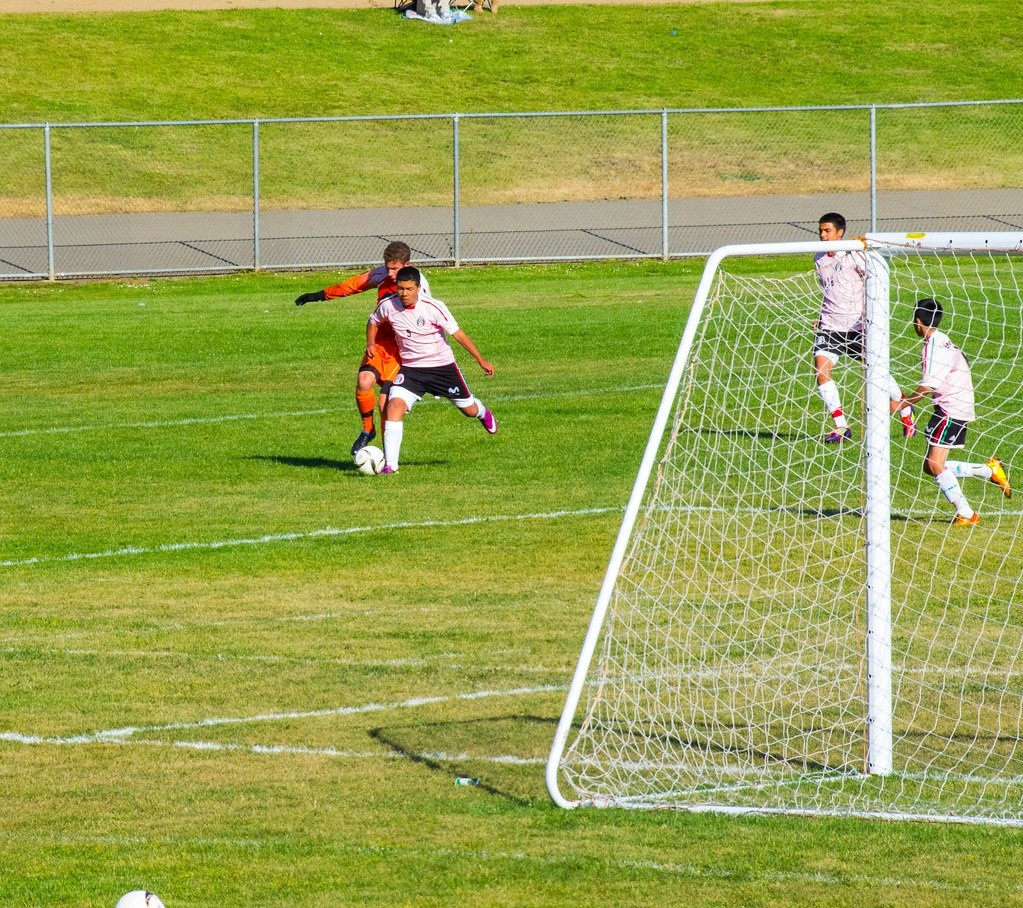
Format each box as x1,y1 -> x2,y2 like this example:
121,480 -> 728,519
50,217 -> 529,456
416,0 -> 451,22
813,213 -> 916,444
365,265 -> 498,475
890,299 -> 1012,526
295,242 -> 430,455
473,0 -> 498,13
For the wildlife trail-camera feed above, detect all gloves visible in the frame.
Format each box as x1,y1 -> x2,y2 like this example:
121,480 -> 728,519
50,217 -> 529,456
295,290 -> 325,306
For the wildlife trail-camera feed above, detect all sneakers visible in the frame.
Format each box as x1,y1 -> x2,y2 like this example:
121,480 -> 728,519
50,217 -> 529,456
951,512 -> 980,525
986,457 -> 1012,498
351,424 -> 376,455
899,404 -> 917,437
824,428 -> 852,443
378,464 -> 399,475
478,406 -> 498,434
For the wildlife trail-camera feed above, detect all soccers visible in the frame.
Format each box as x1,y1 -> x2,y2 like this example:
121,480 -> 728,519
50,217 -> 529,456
353,445 -> 386,476
114,889 -> 166,908
